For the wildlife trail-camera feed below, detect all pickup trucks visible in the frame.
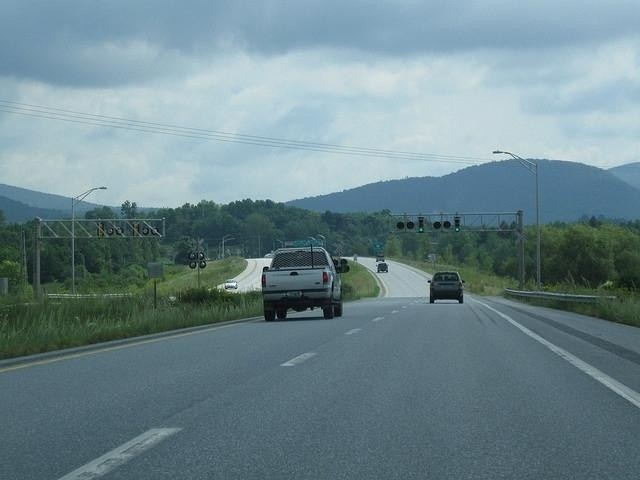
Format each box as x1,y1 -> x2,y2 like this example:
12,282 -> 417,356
261,245 -> 343,321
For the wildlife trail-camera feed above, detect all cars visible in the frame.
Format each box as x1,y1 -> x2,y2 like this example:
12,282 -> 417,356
427,270 -> 465,304
376,262 -> 389,273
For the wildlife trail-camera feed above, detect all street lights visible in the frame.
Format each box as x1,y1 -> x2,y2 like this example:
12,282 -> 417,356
307,234 -> 327,250
217,234 -> 236,259
491,150 -> 541,289
71,186 -> 107,296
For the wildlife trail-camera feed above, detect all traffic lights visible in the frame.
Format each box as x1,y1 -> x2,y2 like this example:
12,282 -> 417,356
454,216 -> 461,233
417,216 -> 424,233
